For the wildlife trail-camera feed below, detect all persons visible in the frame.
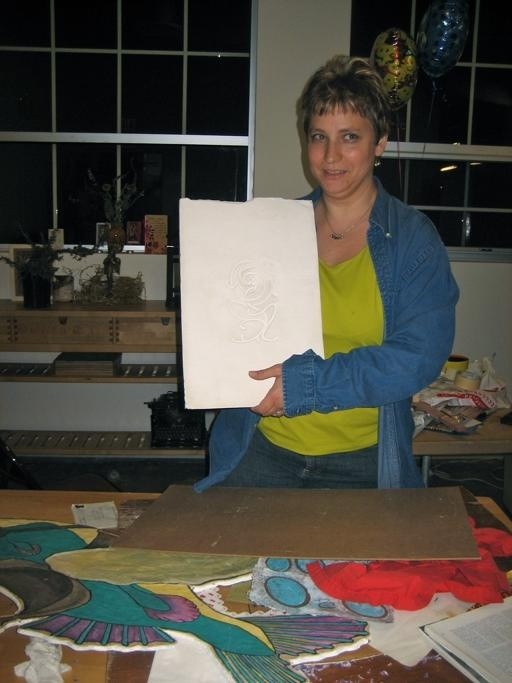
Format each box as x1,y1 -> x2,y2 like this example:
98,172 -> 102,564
191,50 -> 461,496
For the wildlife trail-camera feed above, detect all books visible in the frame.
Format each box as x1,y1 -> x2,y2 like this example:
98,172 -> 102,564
417,605 -> 487,682
424,594 -> 511,682
53,351 -> 125,376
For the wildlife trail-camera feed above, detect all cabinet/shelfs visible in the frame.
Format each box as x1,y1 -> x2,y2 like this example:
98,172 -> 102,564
1,299 -> 208,457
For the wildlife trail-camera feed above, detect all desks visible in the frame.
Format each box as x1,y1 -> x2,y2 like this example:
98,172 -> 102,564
412,391 -> 511,509
0,487 -> 511,682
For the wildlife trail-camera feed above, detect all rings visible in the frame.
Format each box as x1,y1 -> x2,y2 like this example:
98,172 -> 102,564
275,407 -> 284,416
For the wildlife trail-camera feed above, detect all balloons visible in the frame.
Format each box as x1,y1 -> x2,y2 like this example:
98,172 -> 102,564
370,27 -> 419,111
417,0 -> 471,82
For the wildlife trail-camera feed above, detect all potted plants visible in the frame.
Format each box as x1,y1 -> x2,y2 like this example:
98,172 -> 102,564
0,220 -> 107,309
88,168 -> 148,253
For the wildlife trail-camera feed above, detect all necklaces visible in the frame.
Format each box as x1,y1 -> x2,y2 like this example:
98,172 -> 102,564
322,200 -> 372,241
